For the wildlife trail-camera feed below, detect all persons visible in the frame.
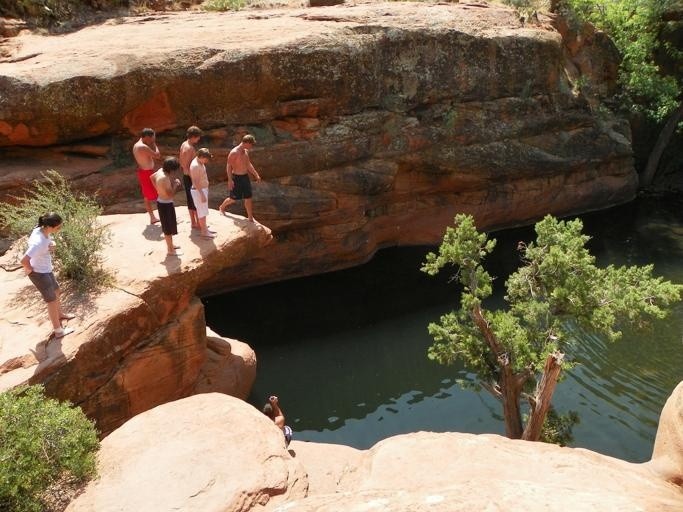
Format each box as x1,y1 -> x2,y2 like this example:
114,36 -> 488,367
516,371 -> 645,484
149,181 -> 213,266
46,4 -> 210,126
19,211 -> 77,339
131,127 -> 181,226
147,156 -> 185,256
186,147 -> 217,238
219,134 -> 261,224
262,396 -> 292,445
179,125 -> 210,229
